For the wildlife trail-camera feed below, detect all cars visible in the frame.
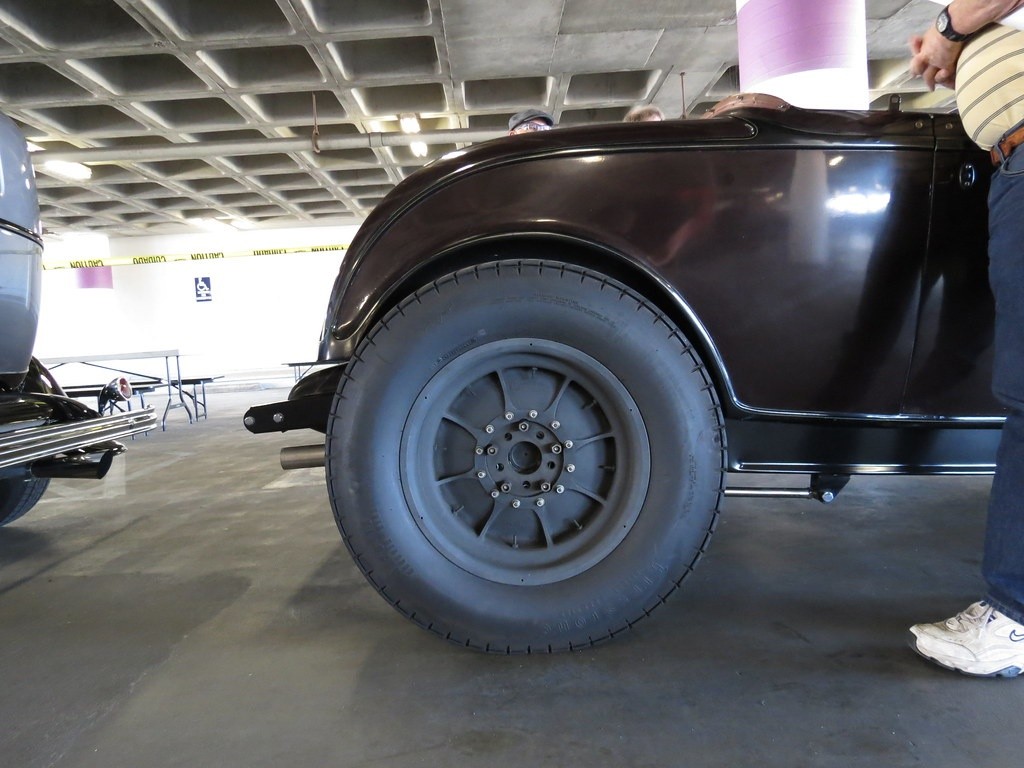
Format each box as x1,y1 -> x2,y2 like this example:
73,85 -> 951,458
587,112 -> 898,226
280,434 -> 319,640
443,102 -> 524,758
241,56 -> 1024,655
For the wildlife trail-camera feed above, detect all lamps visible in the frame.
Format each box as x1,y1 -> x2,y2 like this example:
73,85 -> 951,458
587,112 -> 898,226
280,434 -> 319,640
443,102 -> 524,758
397,113 -> 433,160
21,141 -> 92,182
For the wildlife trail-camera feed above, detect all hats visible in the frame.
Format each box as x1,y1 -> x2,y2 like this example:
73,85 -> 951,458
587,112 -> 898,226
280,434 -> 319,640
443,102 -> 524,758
509,109 -> 554,131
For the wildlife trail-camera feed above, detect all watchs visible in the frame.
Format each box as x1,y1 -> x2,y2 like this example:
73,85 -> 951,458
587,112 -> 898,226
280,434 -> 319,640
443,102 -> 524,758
936,5 -> 973,42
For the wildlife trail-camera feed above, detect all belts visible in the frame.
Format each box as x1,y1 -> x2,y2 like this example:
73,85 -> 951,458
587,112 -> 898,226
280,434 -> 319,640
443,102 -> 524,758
990,126 -> 1024,165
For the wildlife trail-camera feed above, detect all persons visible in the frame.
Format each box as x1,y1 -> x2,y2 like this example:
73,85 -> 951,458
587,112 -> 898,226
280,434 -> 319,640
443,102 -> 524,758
508,109 -> 554,136
623,106 -> 665,122
905,0 -> 1024,678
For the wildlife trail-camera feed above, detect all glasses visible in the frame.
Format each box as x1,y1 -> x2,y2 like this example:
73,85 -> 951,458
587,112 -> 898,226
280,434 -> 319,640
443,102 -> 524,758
512,121 -> 551,132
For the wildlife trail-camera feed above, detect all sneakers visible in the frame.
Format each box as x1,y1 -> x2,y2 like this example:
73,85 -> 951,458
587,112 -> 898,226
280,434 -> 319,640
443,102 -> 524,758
905,600 -> 1024,678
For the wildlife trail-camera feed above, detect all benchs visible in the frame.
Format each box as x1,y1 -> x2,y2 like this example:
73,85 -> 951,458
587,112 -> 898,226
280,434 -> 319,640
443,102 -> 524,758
280,361 -> 352,387
58,380 -> 170,441
62,374 -> 227,421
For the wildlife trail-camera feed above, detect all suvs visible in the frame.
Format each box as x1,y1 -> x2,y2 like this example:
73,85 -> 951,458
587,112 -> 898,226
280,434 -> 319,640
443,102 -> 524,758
0,112 -> 160,529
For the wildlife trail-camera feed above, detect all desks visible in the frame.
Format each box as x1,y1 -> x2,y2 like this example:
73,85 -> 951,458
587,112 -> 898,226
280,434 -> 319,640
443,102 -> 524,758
37,347 -> 194,431
